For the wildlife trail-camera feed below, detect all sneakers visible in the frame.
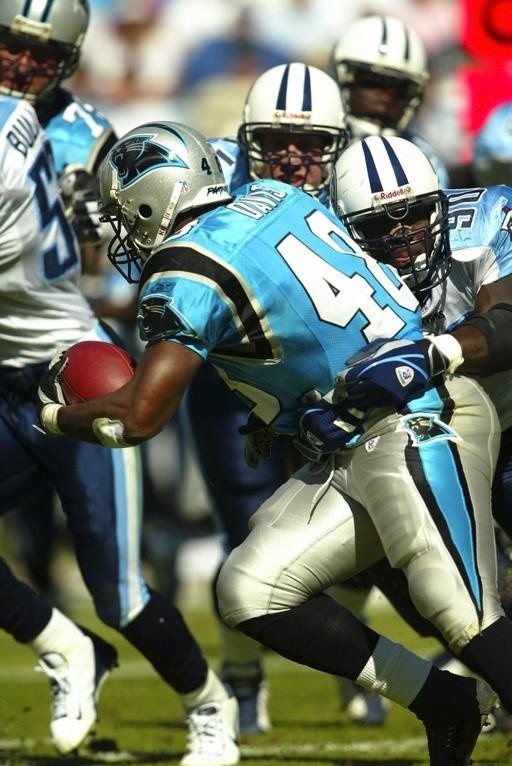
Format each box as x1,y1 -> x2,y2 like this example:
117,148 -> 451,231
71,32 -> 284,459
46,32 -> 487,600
410,665 -> 487,766
46,636 -> 97,756
182,686 -> 244,766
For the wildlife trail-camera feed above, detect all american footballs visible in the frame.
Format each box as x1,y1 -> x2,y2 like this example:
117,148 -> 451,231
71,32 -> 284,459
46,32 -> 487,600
59,341 -> 137,405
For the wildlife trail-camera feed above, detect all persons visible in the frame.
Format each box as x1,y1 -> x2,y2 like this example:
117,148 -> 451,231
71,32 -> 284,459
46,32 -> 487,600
0,0 -> 512,766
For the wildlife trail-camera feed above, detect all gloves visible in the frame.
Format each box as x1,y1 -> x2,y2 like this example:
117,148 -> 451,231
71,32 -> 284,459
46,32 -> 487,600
336,330 -> 448,406
291,385 -> 365,464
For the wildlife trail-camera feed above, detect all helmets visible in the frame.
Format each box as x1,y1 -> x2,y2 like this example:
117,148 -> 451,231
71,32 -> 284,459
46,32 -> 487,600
97,121 -> 230,249
330,134 -> 440,226
335,14 -> 429,85
243,61 -> 347,144
0,0 -> 91,51
